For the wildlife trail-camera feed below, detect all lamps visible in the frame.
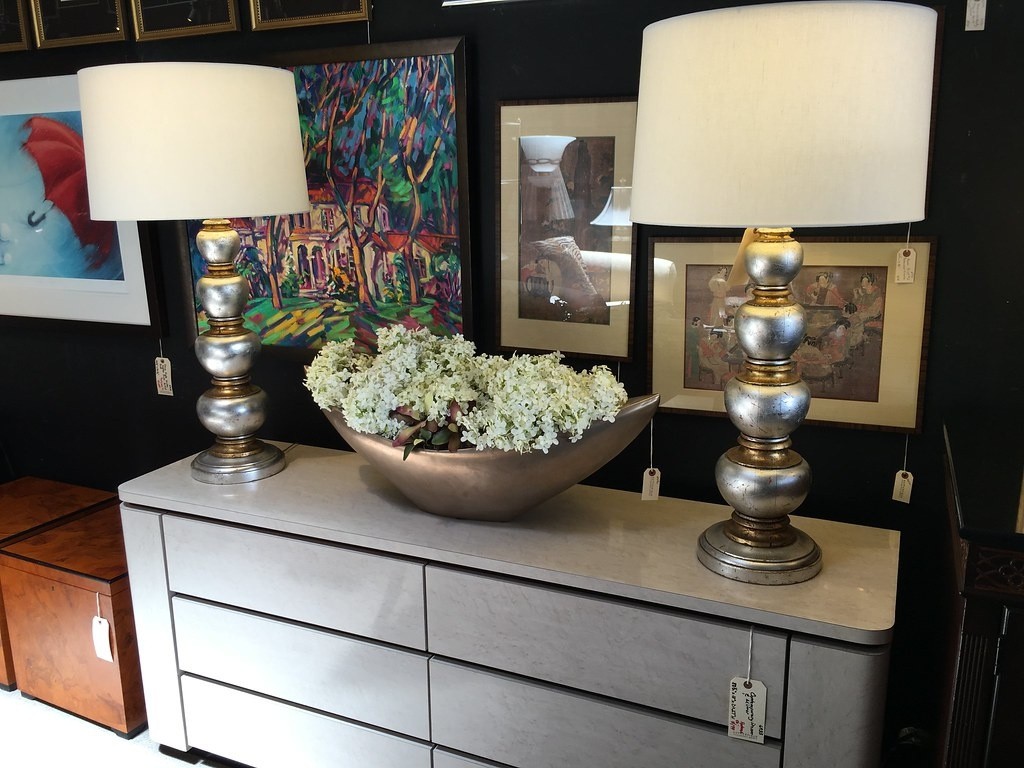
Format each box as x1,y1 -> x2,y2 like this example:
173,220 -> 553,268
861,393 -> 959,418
629,0 -> 938,586
77,62 -> 310,485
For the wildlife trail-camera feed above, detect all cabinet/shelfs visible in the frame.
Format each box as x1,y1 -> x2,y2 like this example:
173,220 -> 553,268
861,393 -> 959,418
118,439 -> 900,768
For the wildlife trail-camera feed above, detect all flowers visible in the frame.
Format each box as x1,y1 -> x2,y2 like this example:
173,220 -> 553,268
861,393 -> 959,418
301,323 -> 628,461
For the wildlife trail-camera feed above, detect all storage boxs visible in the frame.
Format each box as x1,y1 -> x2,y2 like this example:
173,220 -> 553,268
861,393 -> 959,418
0,475 -> 120,692
0,500 -> 148,739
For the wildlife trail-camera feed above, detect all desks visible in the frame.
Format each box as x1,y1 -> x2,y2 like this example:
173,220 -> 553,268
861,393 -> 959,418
921,402 -> 1024,768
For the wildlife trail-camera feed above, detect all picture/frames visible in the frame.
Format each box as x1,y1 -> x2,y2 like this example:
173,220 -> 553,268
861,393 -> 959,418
0,74 -> 151,327
174,34 -> 474,363
248,0 -> 373,32
646,235 -> 938,435
130,0 -> 242,42
30,0 -> 130,49
0,0 -> 33,53
495,95 -> 635,364
